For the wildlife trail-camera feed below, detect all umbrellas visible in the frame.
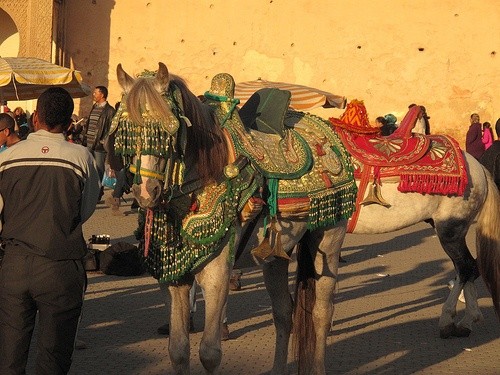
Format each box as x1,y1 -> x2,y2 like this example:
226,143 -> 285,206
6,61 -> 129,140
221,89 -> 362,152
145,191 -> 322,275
235,77 -> 347,110
0,56 -> 91,108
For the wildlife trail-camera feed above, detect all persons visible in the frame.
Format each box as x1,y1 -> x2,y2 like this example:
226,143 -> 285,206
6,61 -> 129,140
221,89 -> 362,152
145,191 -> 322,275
0,87 -> 102,375
375,114 -> 397,135
408,104 -> 427,135
83,85 -> 117,202
466,113 -> 485,165
157,264 -> 243,340
479,118 -> 500,192
108,101 -> 133,217
481,122 -> 495,152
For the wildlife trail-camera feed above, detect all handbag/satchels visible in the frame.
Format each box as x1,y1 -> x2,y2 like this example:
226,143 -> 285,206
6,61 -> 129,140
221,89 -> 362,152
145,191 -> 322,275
99,242 -> 142,276
83,249 -> 99,270
103,176 -> 117,189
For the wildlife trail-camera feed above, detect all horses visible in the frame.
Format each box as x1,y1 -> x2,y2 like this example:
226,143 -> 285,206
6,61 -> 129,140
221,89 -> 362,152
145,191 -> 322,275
117,62 -> 500,375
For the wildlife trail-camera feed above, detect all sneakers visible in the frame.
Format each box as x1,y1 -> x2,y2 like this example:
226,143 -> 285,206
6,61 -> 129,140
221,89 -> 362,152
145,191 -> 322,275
158,314 -> 194,335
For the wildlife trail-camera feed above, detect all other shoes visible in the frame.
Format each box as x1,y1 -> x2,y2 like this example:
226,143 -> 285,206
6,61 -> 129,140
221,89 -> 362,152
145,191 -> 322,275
229,270 -> 243,291
220,321 -> 229,340
97,201 -> 100,204
